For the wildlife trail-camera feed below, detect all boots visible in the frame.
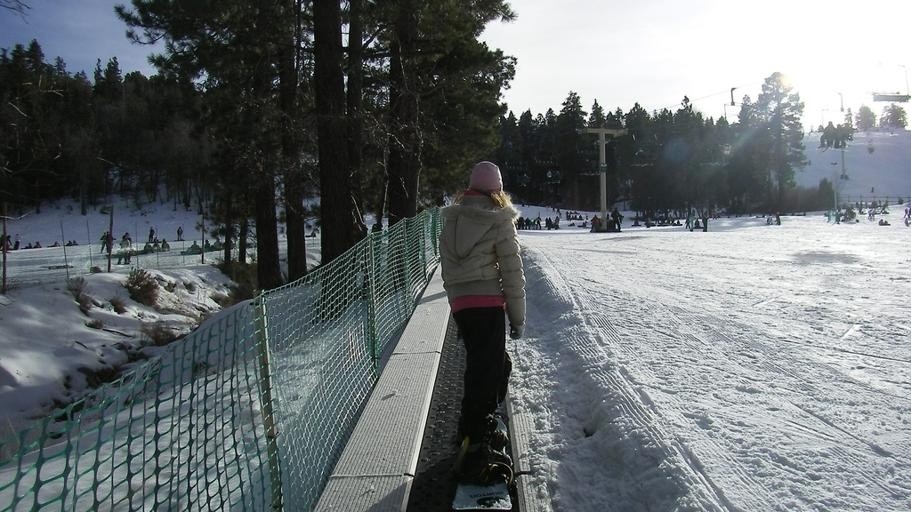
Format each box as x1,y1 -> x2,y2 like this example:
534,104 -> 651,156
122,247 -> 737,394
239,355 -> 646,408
456,415 -> 513,478
469,161 -> 503,194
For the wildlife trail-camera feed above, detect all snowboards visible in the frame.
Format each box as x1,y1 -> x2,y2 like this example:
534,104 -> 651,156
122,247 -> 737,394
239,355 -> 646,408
452,416 -> 512,511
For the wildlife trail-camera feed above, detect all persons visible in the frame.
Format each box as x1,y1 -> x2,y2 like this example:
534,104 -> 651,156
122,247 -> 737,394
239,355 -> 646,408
767,215 -> 774,225
437,160 -> 529,488
517,199 -> 723,232
1,216 -> 223,266
824,196 -> 911,227
774,211 -> 782,225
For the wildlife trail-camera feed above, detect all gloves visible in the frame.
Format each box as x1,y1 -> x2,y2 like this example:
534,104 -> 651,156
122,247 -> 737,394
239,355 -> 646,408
509,322 -> 525,340
